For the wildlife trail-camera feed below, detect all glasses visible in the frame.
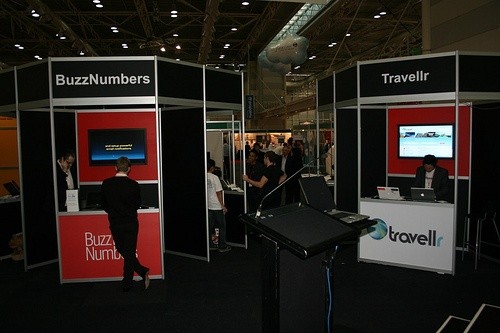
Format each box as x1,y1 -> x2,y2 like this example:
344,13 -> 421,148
65,160 -> 74,164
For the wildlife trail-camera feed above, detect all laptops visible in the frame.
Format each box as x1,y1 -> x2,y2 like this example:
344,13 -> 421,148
4,182 -> 21,197
410,188 -> 447,204
298,176 -> 370,224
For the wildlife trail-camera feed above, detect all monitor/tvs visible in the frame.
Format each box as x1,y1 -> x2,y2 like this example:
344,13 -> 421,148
88,128 -> 147,165
397,123 -> 456,159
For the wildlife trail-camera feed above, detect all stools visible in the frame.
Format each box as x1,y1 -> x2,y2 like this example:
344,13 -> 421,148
461,207 -> 500,270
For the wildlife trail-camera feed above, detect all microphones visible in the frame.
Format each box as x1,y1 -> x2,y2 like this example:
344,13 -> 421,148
255,159 -> 316,218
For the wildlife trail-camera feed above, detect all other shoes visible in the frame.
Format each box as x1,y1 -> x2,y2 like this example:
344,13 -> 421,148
209,242 -> 219,251
141,268 -> 150,290
123,282 -> 139,292
218,245 -> 232,252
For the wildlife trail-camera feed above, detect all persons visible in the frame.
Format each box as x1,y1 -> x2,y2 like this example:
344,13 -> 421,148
100,157 -> 150,293
49,149 -> 77,212
323,139 -> 331,154
207,159 -> 232,253
224,138 -> 304,211
415,155 -> 448,203
